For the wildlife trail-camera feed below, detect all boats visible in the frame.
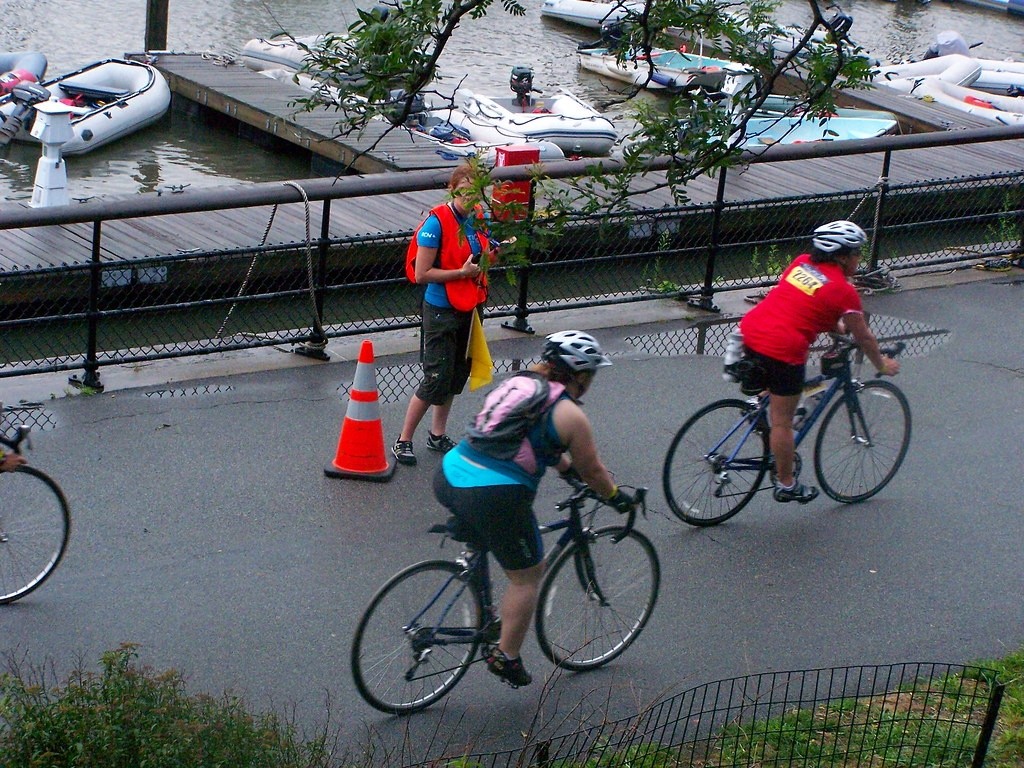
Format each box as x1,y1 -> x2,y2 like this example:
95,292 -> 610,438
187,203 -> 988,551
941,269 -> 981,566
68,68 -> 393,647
0,58 -> 174,158
251,68 -> 389,126
451,65 -> 622,161
538,0 -> 1024,146
370,88 -> 565,172
236,29 -> 442,99
0,51 -> 47,110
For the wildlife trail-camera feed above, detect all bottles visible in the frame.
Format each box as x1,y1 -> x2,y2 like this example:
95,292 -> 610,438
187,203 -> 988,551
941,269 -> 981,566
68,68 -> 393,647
790,389 -> 825,433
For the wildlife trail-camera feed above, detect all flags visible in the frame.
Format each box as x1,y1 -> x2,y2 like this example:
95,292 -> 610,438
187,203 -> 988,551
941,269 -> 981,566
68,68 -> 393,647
468,308 -> 493,392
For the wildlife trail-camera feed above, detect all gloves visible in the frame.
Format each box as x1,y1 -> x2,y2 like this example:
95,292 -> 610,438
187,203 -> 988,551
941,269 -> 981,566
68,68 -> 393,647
604,484 -> 635,514
558,461 -> 583,487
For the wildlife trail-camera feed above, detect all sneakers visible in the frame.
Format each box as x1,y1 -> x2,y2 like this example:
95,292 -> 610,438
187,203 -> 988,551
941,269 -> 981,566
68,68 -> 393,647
390,434 -> 418,466
740,402 -> 769,433
772,479 -> 820,503
426,429 -> 458,454
485,646 -> 534,686
476,612 -> 504,641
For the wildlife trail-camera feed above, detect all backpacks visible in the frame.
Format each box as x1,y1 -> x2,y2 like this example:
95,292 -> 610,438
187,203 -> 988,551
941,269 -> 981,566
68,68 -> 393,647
462,367 -> 566,462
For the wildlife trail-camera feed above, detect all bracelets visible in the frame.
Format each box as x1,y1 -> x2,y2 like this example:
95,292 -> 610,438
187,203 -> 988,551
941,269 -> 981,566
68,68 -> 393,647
602,484 -> 618,499
494,247 -> 498,255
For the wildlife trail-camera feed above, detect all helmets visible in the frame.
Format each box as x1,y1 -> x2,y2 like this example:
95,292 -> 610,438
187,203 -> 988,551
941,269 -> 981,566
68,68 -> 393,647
812,219 -> 869,253
540,330 -> 612,373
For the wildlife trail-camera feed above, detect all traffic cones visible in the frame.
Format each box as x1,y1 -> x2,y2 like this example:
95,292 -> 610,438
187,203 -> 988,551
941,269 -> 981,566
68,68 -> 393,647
320,339 -> 402,481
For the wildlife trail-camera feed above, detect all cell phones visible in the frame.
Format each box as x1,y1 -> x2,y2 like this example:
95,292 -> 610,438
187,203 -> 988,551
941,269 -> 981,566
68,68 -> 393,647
472,253 -> 482,264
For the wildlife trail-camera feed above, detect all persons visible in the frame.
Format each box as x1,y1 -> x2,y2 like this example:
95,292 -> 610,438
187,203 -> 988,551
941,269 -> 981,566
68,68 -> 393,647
391,164 -> 516,464
431,328 -> 635,687
739,221 -> 900,502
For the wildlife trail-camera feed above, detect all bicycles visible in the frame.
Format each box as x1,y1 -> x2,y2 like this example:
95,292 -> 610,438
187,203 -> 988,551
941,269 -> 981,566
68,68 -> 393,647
350,466 -> 663,719
0,402 -> 87,612
658,337 -> 915,532
